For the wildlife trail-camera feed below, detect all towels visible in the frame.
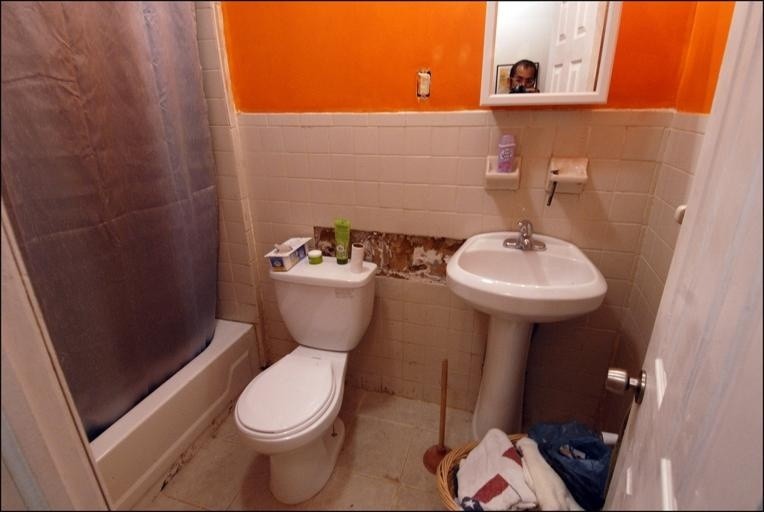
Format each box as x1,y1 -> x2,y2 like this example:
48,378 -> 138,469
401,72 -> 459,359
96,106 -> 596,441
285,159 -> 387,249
455,428 -> 536,510
506,435 -> 588,510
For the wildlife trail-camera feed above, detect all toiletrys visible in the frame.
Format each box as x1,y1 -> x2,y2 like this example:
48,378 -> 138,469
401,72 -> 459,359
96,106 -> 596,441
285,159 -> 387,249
307,249 -> 323,263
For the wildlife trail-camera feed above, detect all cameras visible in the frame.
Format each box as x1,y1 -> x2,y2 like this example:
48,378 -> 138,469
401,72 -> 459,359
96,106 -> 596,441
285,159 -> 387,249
510,84 -> 526,93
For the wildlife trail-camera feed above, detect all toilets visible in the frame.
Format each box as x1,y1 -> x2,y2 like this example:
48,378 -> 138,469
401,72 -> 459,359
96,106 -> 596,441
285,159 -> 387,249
234,257 -> 377,503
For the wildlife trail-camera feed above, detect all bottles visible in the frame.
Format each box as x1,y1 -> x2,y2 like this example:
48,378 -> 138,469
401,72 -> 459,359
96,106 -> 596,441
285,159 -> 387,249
495,133 -> 516,173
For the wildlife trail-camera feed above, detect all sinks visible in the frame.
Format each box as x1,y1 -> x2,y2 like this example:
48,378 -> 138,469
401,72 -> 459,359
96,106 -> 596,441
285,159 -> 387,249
446,231 -> 608,323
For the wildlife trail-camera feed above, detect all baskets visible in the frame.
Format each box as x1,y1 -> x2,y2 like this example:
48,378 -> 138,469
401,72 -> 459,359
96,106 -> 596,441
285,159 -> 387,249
437,433 -> 526,511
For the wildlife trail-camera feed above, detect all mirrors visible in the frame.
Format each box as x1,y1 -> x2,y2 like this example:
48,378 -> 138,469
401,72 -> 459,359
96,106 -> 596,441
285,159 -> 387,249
478,1 -> 624,106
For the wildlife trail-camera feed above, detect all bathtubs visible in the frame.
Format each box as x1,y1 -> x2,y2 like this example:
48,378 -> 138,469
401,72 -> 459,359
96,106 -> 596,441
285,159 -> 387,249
89,317 -> 259,512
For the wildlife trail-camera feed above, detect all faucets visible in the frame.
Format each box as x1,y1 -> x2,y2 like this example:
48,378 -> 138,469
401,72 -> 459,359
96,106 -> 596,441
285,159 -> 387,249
502,221 -> 546,252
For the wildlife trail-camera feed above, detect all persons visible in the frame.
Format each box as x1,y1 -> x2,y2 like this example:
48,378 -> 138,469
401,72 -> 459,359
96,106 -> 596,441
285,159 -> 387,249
505,59 -> 540,93
